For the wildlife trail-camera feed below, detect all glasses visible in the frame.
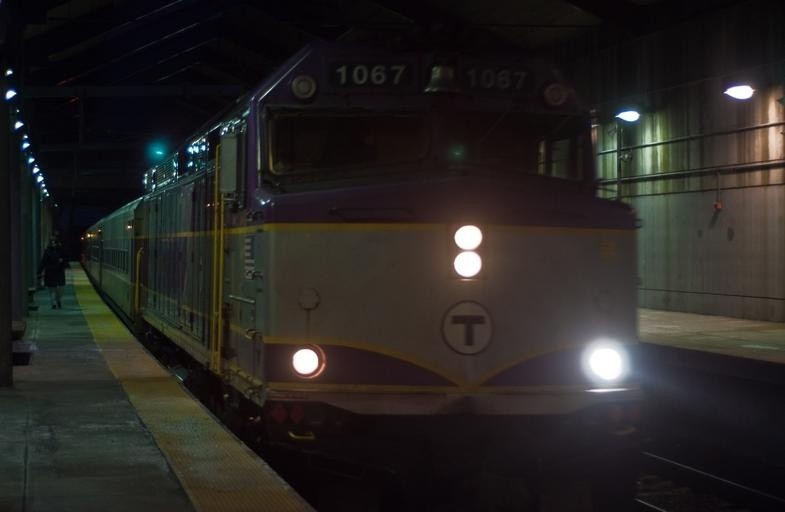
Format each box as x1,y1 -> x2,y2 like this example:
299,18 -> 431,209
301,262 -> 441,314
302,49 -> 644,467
721,73 -> 757,100
2,68 -> 50,199
613,102 -> 643,122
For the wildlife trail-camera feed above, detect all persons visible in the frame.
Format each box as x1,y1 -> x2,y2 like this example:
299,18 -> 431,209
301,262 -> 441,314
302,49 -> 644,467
57,240 -> 66,296
37,237 -> 65,309
